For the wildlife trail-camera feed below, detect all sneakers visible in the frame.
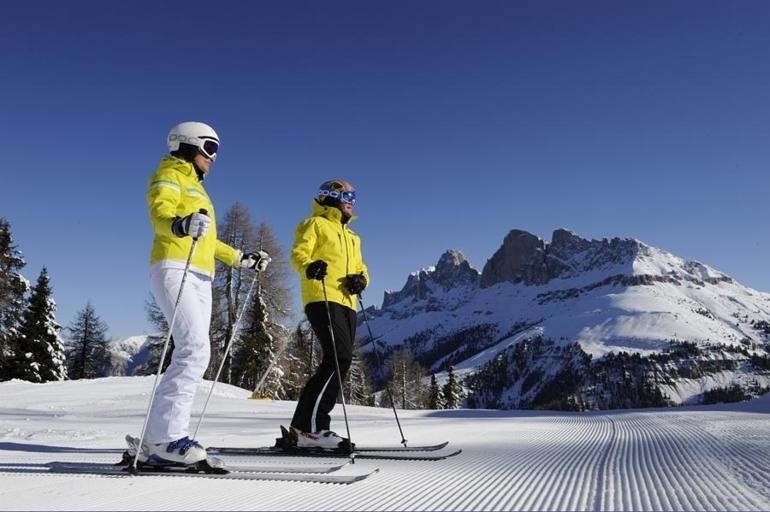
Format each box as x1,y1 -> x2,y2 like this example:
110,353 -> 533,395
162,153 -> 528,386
124,433 -> 208,468
281,423 -> 350,452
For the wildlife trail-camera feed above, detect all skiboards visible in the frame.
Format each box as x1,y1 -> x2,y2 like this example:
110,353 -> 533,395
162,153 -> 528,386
205,442 -> 462,460
44,460 -> 379,484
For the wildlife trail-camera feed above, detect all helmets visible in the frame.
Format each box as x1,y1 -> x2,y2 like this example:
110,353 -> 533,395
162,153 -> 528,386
167,121 -> 221,154
318,178 -> 356,203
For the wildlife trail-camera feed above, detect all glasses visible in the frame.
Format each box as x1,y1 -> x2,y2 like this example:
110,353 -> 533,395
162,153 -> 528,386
318,189 -> 357,208
167,133 -> 220,163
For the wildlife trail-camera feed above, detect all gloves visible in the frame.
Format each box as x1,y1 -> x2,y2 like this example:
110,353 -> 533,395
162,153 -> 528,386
306,259 -> 328,280
239,249 -> 273,273
174,212 -> 212,239
344,273 -> 367,296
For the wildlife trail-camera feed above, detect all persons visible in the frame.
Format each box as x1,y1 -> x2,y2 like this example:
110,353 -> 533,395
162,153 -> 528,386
132,120 -> 273,473
276,177 -> 371,454
